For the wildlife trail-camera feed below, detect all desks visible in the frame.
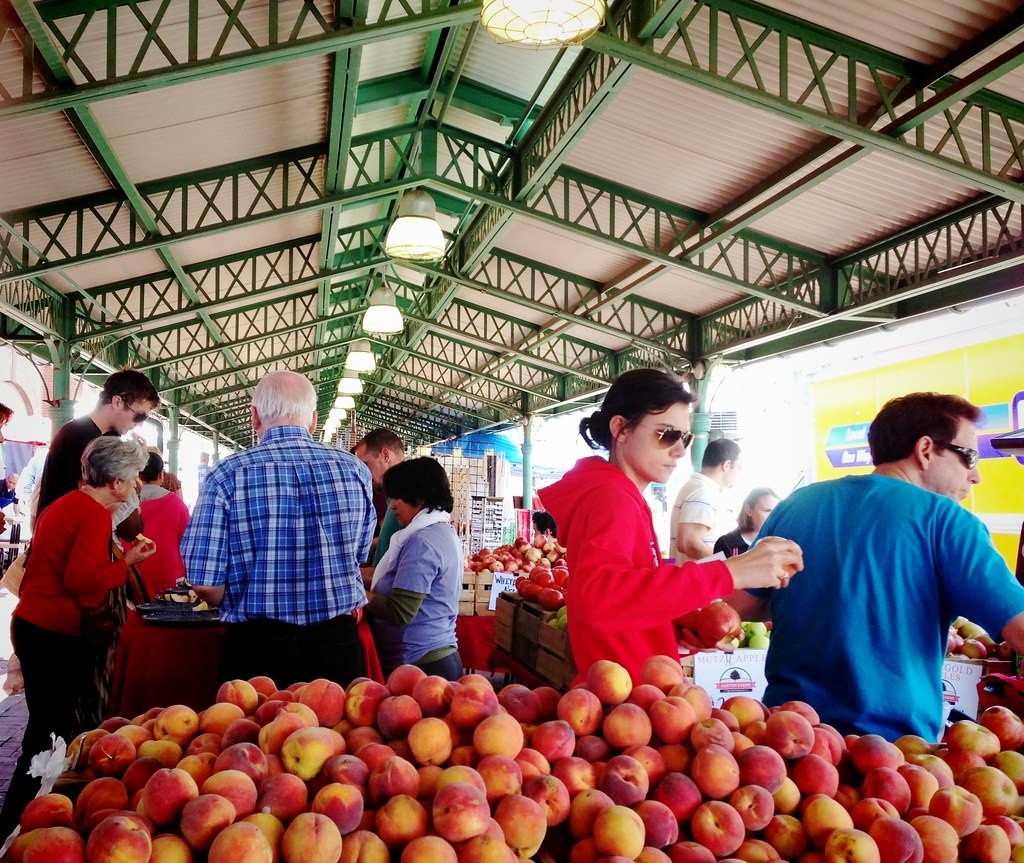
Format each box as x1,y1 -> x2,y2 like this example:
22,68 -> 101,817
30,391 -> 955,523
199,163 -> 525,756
117,598 -> 389,739
482,645 -> 551,690
457,616 -> 516,686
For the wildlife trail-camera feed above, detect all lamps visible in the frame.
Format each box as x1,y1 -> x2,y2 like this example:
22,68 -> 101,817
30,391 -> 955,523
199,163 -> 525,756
319,425 -> 336,442
325,418 -> 342,427
477,0 -> 607,52
329,408 -> 348,420
384,185 -> 448,265
337,369 -> 365,395
335,395 -> 356,411
363,266 -> 405,337
344,339 -> 378,375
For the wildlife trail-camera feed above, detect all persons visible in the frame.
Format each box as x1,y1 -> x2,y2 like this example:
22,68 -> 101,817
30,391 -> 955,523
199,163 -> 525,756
112,446 -> 190,601
180,371 -> 377,689
198,452 -> 211,491
0,473 -> 19,509
669,439 -> 741,566
364,456 -> 464,682
532,512 -> 556,539
714,486 -> 783,559
538,369 -> 803,691
0,437 -> 156,848
16,446 -> 49,534
35,370 -> 158,519
350,428 -> 404,581
724,391 -> 1024,744
0,403 -> 13,443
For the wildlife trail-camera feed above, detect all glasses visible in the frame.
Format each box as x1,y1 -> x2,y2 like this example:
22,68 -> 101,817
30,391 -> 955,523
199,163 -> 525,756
937,441 -> 980,470
638,422 -> 693,449
124,402 -> 147,425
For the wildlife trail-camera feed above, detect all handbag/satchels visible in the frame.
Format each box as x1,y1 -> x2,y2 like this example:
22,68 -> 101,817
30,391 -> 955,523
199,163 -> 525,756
1,546 -> 31,598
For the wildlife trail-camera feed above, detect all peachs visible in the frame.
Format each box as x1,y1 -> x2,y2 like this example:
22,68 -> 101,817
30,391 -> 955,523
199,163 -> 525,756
10,655 -> 1024,863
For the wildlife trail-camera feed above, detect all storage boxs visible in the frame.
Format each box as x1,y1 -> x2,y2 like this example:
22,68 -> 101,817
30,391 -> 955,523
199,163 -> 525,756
492,590 -> 520,649
944,658 -> 1015,726
692,650 -> 767,709
530,608 -> 571,686
460,571 -> 473,614
476,572 -> 520,617
513,600 -> 555,671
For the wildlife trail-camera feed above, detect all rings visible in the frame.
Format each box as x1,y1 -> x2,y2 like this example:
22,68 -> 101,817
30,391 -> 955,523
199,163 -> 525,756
780,572 -> 788,580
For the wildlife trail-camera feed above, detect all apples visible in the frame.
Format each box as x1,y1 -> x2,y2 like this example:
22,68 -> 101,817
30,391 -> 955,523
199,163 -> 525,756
515,566 -> 772,650
464,534 -> 567,573
947,616 -> 1013,661
136,533 -> 154,543
164,590 -> 208,611
